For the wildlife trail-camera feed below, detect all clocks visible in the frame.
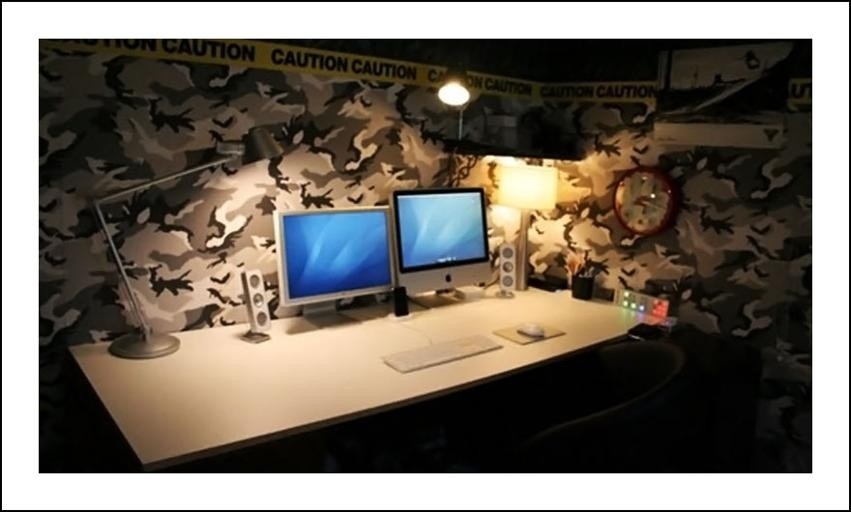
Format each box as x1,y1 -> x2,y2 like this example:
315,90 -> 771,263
613,166 -> 677,235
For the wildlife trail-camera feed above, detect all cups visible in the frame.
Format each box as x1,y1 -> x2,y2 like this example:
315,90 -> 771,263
572,276 -> 594,300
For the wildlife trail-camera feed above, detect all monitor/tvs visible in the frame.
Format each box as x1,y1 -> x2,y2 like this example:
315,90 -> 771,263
273,207 -> 390,325
390,187 -> 491,311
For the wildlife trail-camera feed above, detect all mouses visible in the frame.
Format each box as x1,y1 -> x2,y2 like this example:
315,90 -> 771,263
517,323 -> 546,338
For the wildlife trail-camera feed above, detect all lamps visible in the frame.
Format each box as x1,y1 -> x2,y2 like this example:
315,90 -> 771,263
435,64 -> 479,154
91,126 -> 296,358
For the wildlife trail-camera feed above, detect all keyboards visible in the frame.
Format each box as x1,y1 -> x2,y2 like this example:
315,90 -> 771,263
382,332 -> 504,374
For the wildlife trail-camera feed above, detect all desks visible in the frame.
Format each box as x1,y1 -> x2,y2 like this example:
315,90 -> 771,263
66,281 -> 687,473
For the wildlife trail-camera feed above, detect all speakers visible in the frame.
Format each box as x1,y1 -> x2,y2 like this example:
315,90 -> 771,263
241,269 -> 270,342
498,243 -> 517,300
